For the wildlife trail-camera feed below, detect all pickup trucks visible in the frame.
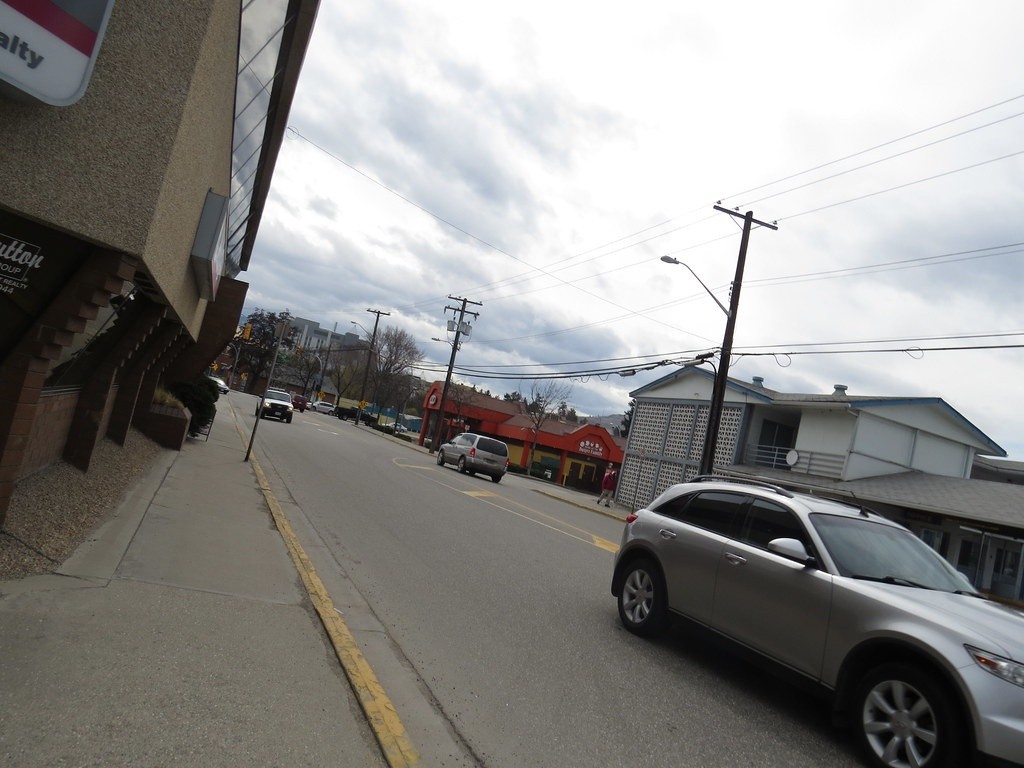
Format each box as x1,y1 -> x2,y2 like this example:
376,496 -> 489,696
332,406 -> 378,426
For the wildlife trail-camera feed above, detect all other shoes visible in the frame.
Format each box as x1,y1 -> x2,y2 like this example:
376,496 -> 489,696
597,499 -> 600,504
605,504 -> 610,507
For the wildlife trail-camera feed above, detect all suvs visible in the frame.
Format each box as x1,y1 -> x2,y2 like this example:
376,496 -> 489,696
437,432 -> 511,484
611,474 -> 1024,768
255,387 -> 295,424
289,392 -> 308,412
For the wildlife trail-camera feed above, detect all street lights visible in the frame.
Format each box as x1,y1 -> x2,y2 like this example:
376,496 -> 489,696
429,337 -> 457,454
228,342 -> 243,389
660,255 -> 736,482
350,321 -> 375,424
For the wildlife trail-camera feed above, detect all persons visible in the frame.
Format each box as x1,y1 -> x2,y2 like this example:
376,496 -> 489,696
597,471 -> 617,508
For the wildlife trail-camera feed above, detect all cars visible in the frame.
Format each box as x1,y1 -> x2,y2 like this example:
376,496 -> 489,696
382,422 -> 407,433
208,375 -> 230,395
305,401 -> 337,416
